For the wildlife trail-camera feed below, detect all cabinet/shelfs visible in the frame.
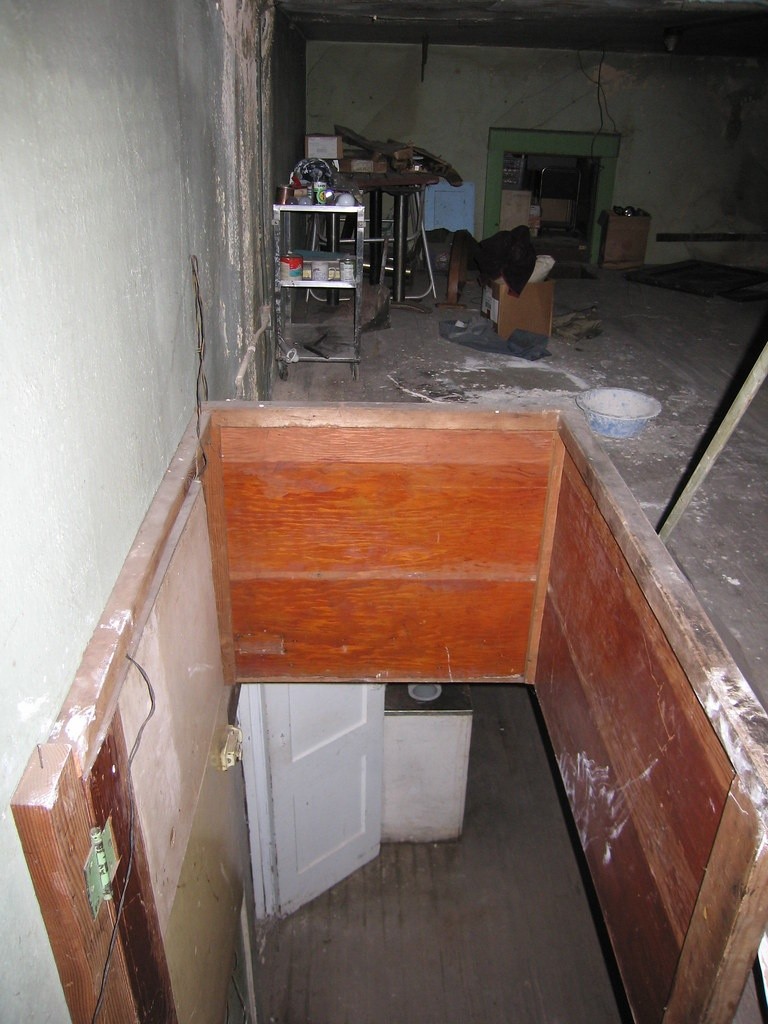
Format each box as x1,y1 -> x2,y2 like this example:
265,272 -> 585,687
272,205 -> 365,381
597,209 -> 652,270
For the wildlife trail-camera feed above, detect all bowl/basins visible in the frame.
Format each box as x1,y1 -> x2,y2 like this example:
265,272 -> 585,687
576,387 -> 662,439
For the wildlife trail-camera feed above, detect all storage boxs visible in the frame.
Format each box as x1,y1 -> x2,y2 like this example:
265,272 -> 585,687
303,133 -> 389,174
480,275 -> 555,339
540,198 -> 571,222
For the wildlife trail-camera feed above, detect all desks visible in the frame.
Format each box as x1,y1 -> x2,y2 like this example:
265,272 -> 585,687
303,173 -> 440,306
